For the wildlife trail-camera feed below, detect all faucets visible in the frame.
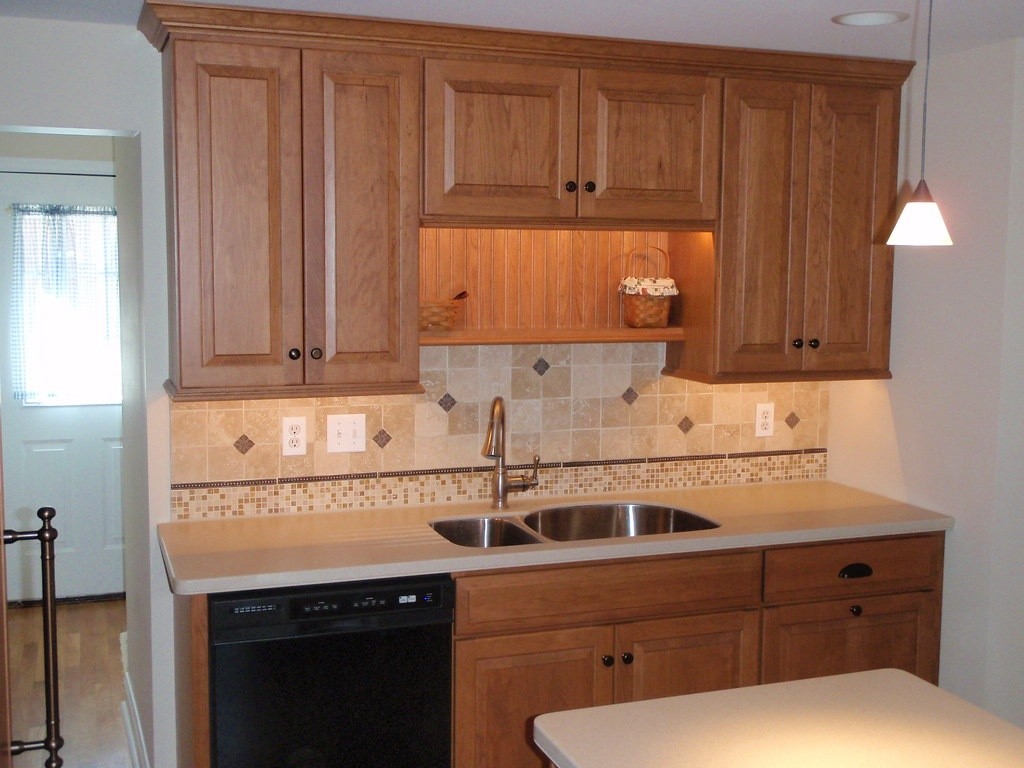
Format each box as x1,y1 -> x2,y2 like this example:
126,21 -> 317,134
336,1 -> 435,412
480,396 -> 512,510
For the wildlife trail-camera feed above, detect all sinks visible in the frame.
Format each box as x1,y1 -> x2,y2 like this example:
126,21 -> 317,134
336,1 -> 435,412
426,515 -> 545,548
522,502 -> 722,543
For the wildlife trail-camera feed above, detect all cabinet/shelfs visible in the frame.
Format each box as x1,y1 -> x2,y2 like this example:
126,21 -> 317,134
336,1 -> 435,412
191,530 -> 947,768
135,0 -> 917,404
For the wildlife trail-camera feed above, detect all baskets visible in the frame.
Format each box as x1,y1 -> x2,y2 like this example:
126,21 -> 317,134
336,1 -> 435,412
617,245 -> 679,327
419,300 -> 466,330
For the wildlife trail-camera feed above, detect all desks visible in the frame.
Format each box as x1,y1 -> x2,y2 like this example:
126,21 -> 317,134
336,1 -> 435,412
533,667 -> 1024,768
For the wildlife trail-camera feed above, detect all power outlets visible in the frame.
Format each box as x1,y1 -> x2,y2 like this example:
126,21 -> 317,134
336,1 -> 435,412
757,403 -> 775,437
283,416 -> 307,455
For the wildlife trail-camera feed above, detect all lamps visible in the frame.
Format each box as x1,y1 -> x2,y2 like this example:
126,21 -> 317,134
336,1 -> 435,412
885,0 -> 954,245
